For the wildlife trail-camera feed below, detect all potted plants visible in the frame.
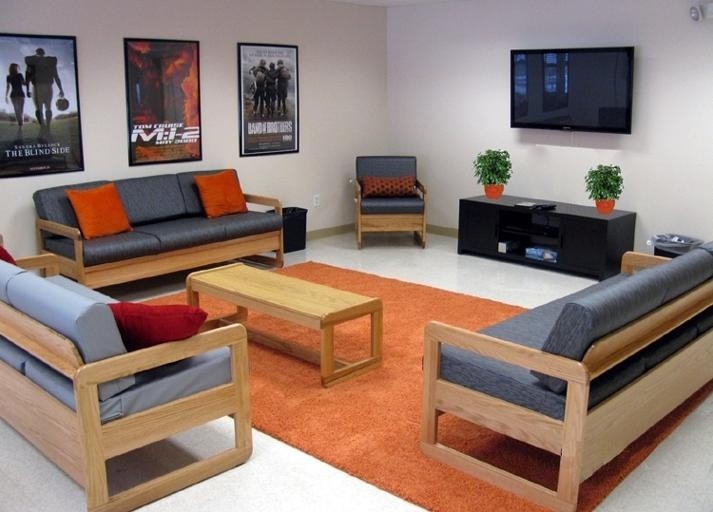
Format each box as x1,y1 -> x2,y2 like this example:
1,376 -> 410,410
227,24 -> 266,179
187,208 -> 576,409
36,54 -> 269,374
473,147 -> 512,198
583,164 -> 624,216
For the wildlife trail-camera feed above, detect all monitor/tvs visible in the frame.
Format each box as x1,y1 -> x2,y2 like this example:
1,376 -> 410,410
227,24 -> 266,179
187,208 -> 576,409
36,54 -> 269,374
511,47 -> 634,134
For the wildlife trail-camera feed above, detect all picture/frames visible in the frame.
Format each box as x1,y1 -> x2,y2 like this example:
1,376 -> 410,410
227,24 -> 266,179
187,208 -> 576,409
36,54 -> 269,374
236,42 -> 300,157
0,32 -> 84,179
123,37 -> 203,168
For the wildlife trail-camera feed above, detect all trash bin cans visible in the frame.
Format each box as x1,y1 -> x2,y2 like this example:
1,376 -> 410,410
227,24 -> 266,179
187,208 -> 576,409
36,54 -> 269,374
651,233 -> 705,259
268,206 -> 308,254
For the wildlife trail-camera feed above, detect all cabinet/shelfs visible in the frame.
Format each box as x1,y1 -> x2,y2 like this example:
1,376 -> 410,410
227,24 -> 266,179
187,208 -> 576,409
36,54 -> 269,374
458,193 -> 636,283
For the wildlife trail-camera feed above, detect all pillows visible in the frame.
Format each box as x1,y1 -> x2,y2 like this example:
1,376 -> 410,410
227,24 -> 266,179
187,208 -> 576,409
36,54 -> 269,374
106,302 -> 208,350
193,170 -> 249,220
361,175 -> 416,198
64,182 -> 133,240
0,243 -> 18,266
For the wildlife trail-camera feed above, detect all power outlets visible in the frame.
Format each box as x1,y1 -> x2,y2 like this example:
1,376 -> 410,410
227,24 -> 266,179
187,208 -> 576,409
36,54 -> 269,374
313,194 -> 320,208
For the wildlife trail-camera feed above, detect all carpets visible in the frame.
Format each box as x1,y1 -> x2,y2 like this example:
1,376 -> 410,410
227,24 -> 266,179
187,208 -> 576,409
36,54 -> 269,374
138,260 -> 713,512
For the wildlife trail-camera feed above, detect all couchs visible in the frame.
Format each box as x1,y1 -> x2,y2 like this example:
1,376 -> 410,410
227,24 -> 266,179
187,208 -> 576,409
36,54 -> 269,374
419,250 -> 713,512
0,255 -> 253,512
32,169 -> 284,290
353,155 -> 427,250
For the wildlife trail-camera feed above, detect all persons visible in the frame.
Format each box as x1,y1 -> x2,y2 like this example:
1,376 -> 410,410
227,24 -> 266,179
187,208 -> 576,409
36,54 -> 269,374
3,62 -> 28,126
250,66 -> 268,117
266,61 -> 279,116
257,58 -> 267,69
23,48 -> 65,143
275,60 -> 291,116
